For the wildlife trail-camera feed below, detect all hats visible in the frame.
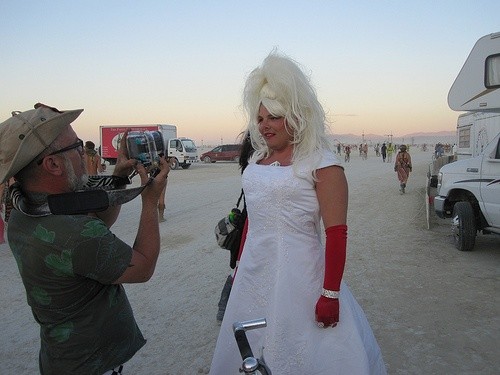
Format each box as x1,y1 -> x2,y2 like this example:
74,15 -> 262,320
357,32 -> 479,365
0,103 -> 84,184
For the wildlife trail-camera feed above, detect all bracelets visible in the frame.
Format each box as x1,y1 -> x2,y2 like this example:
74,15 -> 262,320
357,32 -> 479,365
321,288 -> 340,299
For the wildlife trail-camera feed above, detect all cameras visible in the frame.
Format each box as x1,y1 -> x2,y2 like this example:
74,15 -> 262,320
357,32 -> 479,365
126,130 -> 164,172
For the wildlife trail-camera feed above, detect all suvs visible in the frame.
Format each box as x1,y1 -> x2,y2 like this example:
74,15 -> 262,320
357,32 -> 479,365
199,144 -> 245,165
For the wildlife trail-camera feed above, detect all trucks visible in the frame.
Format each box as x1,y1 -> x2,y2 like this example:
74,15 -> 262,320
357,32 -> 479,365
432,32 -> 500,250
100,124 -> 199,169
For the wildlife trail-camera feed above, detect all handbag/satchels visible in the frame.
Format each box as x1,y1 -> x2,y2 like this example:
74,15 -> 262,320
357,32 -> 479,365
213,192 -> 248,251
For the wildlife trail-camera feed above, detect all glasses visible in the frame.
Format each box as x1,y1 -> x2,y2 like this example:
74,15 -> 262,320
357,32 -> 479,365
37,137 -> 84,166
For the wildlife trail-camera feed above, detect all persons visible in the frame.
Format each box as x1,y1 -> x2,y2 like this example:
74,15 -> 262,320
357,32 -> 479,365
394,145 -> 413,194
333,143 -> 457,163
232,125 -> 264,287
84,141 -> 107,180
1,101 -> 169,375
0,173 -> 16,228
158,177 -> 166,222
207,54 -> 389,374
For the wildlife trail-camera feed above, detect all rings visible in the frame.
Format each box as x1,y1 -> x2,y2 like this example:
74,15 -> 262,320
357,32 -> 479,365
329,322 -> 337,328
318,321 -> 324,328
